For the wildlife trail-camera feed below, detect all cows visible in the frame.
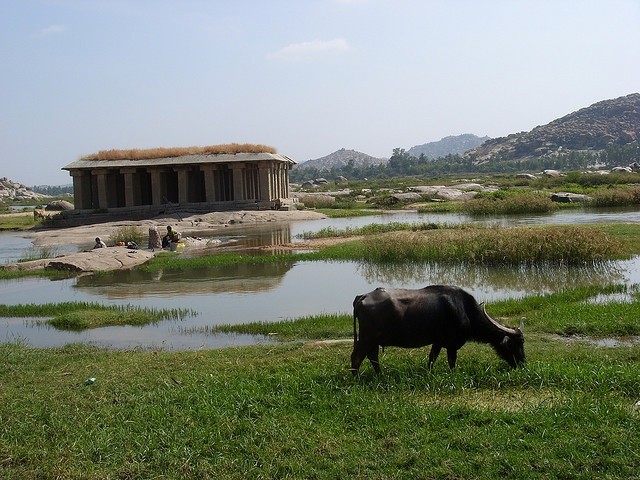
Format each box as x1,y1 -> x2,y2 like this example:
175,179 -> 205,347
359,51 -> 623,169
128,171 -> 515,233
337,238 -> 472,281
351,285 -> 526,383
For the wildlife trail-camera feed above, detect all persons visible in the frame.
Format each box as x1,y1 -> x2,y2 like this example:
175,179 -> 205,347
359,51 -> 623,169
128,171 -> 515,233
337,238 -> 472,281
163,225 -> 182,243
93,237 -> 107,248
